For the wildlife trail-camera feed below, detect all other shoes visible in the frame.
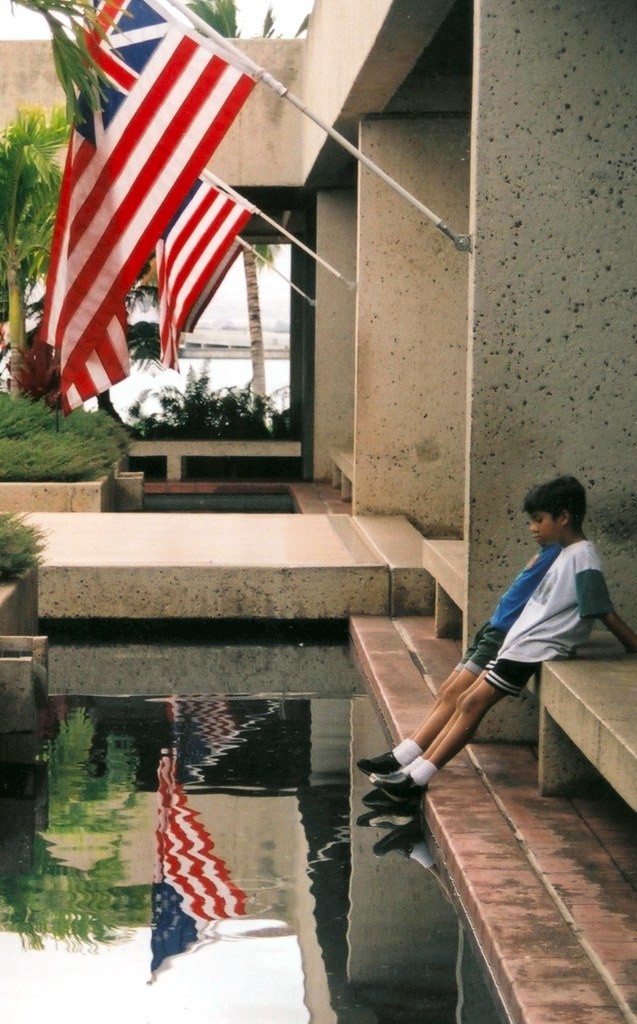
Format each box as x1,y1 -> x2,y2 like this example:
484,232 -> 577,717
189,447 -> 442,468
369,772 -> 429,803
356,809 -> 417,829
362,790 -> 420,810
373,819 -> 423,856
357,751 -> 401,776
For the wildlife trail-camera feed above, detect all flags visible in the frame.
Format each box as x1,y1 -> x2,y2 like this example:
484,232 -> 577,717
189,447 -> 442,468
40,0 -> 258,417
152,693 -> 245,987
155,177 -> 253,373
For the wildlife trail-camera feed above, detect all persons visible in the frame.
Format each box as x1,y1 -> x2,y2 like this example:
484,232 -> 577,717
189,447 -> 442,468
358,477 -> 637,804
354,809 -> 442,904
